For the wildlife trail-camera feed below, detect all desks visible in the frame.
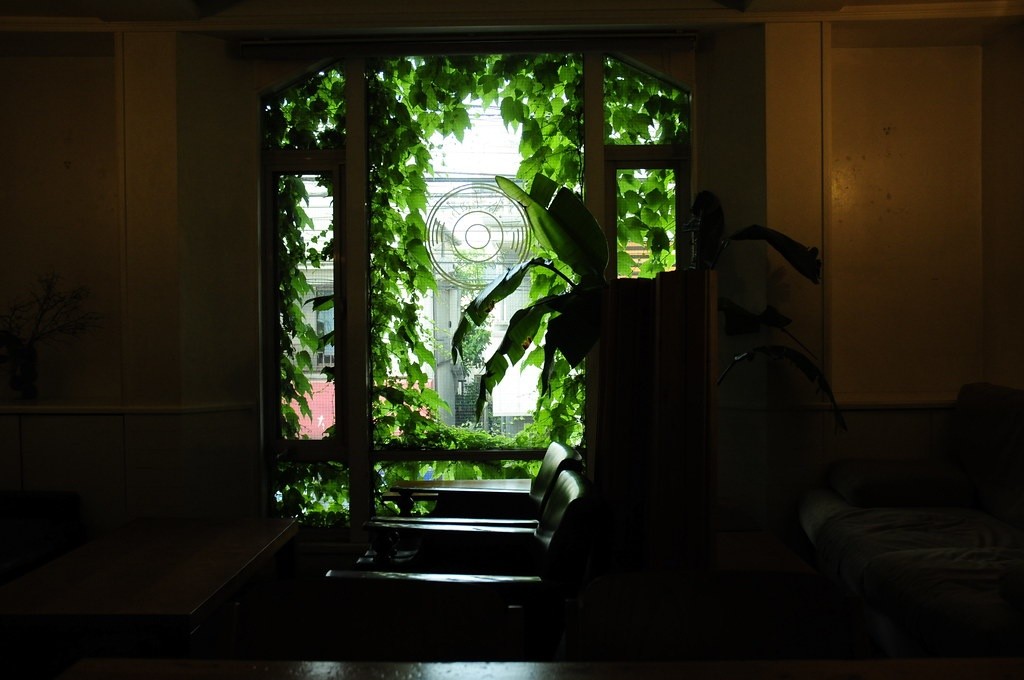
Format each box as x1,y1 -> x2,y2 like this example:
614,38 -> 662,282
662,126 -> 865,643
0,510 -> 306,657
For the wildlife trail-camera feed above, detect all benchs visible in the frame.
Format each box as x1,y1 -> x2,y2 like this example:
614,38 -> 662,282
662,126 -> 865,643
324,266 -> 841,662
800,383 -> 1024,660
0,487 -> 90,588
